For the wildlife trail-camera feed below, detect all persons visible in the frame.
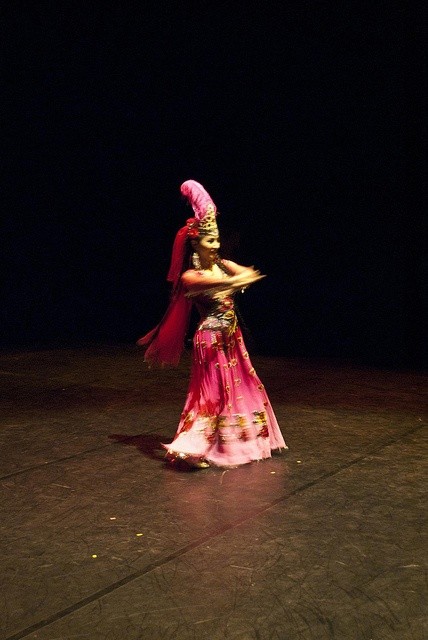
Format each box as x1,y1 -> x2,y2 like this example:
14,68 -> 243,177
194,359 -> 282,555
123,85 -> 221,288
164,221 -> 290,471
